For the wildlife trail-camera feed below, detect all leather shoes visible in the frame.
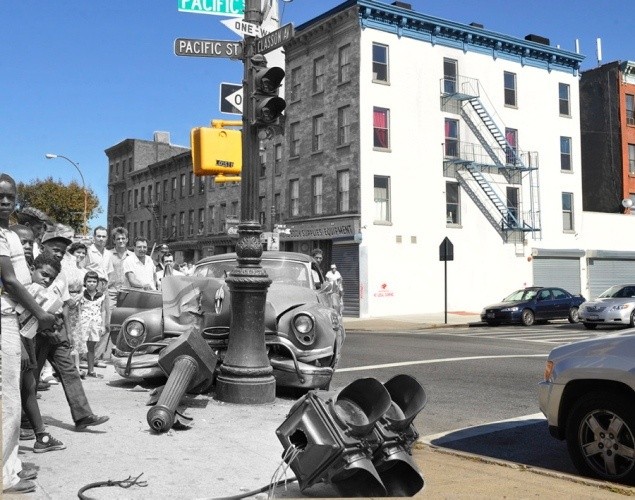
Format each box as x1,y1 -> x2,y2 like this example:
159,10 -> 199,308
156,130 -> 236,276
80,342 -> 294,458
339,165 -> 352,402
3,479 -> 36,494
20,428 -> 35,440
76,414 -> 109,430
17,470 -> 37,480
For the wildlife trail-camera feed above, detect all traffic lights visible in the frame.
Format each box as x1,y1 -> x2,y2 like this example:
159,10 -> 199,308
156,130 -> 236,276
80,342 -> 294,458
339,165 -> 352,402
251,66 -> 286,127
276,374 -> 428,497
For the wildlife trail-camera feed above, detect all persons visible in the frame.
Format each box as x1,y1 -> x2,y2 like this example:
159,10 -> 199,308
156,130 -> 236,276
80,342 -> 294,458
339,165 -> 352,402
10,223 -> 33,262
68,242 -> 87,380
152,244 -> 169,272
28,252 -> 61,287
82,226 -> 112,367
108,226 -> 134,316
35,318 -> 109,430
122,236 -> 160,291
37,230 -> 71,261
156,251 -> 186,287
20,341 -> 67,454
0,174 -> 64,495
180,259 -> 197,271
78,271 -> 105,377
325,264 -> 344,314
299,248 -> 324,282
17,207 -> 43,256
34,358 -> 59,398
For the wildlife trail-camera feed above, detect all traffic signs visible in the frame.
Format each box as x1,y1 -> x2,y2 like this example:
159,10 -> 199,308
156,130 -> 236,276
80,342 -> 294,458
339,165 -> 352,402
220,17 -> 286,52
177,0 -> 245,18
174,38 -> 244,59
219,83 -> 243,115
253,23 -> 294,55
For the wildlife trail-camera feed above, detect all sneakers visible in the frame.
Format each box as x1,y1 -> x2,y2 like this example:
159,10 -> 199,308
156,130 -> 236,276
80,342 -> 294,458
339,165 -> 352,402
87,373 -> 104,379
37,381 -> 51,391
34,432 -> 67,453
78,370 -> 86,380
94,362 -> 106,367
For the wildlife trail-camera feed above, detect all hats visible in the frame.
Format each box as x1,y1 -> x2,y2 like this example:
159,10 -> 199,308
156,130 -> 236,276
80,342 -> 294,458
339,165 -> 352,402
331,264 -> 337,268
41,225 -> 73,245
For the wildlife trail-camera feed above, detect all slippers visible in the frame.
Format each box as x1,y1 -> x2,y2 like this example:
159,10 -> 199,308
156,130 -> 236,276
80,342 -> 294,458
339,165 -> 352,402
44,376 -> 57,384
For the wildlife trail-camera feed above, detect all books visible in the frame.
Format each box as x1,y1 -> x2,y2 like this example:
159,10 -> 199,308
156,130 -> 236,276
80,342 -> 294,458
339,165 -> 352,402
16,282 -> 62,340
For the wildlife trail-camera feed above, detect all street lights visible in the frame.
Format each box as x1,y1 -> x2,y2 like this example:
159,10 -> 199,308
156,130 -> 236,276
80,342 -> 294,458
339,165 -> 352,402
46,154 -> 88,236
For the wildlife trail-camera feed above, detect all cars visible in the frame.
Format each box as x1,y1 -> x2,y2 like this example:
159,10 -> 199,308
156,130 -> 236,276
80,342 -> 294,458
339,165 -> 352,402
578,283 -> 635,329
103,251 -> 346,391
536,328 -> 634,482
481,287 -> 585,326
